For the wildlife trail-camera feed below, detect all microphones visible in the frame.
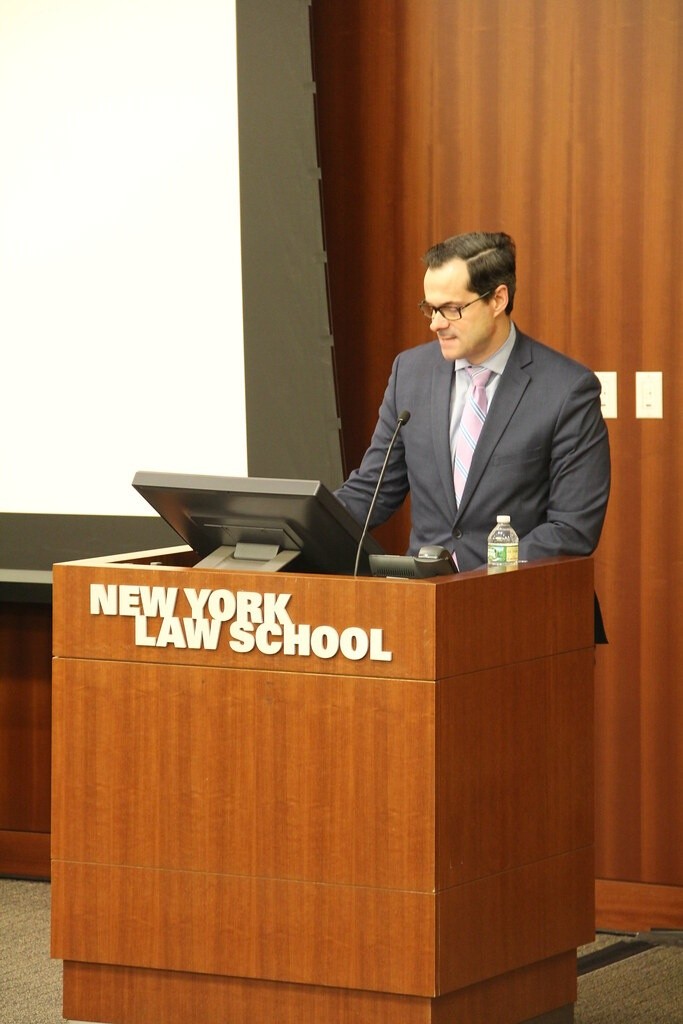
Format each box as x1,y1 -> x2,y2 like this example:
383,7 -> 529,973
354,410 -> 411,579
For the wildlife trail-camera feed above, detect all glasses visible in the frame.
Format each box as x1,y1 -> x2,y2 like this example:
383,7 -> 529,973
419,290 -> 491,320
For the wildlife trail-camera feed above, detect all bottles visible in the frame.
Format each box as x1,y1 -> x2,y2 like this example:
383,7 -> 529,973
488,515 -> 519,567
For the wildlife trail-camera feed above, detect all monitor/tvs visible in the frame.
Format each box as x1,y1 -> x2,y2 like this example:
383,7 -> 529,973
132,471 -> 387,578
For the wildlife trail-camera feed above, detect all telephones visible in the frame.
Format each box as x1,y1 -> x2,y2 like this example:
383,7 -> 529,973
368,544 -> 459,578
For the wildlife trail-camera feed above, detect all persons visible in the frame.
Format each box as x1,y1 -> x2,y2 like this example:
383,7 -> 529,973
330,229 -> 611,646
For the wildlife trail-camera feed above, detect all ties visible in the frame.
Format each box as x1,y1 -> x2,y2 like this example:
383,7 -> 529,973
452,365 -> 492,511
